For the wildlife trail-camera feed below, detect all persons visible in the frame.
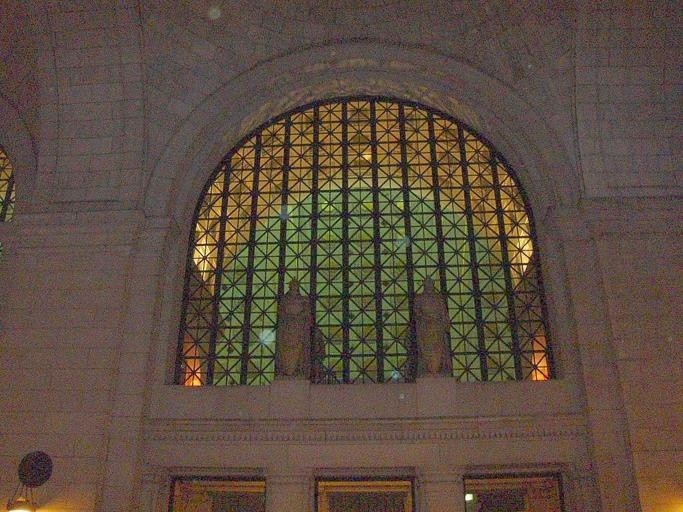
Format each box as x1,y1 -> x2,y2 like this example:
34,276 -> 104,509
274,277 -> 313,376
412,274 -> 452,375
